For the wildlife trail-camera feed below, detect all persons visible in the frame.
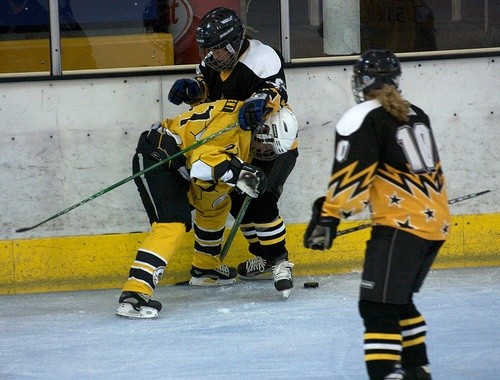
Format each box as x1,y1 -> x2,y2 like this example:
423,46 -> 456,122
302,48 -> 453,380
167,6 -> 300,299
114,99 -> 298,319
143,0 -> 202,65
0,0 -> 97,73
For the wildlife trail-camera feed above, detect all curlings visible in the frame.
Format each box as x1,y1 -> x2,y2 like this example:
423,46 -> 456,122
303,280 -> 320,291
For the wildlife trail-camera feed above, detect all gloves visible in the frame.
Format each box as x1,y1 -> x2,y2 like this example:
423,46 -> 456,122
302,208 -> 340,251
236,93 -> 272,131
226,162 -> 271,199
167,78 -> 203,105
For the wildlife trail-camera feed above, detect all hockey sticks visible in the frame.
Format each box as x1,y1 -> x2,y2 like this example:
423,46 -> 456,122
174,195 -> 256,286
336,189 -> 494,238
15,105 -> 275,233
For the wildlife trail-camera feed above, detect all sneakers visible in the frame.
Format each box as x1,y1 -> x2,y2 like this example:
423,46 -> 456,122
235,257 -> 273,280
188,264 -> 238,287
272,260 -> 294,300
115,290 -> 163,320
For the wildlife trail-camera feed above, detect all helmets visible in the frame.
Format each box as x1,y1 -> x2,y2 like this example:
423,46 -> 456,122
254,103 -> 299,155
193,6 -> 244,48
353,50 -> 402,92
